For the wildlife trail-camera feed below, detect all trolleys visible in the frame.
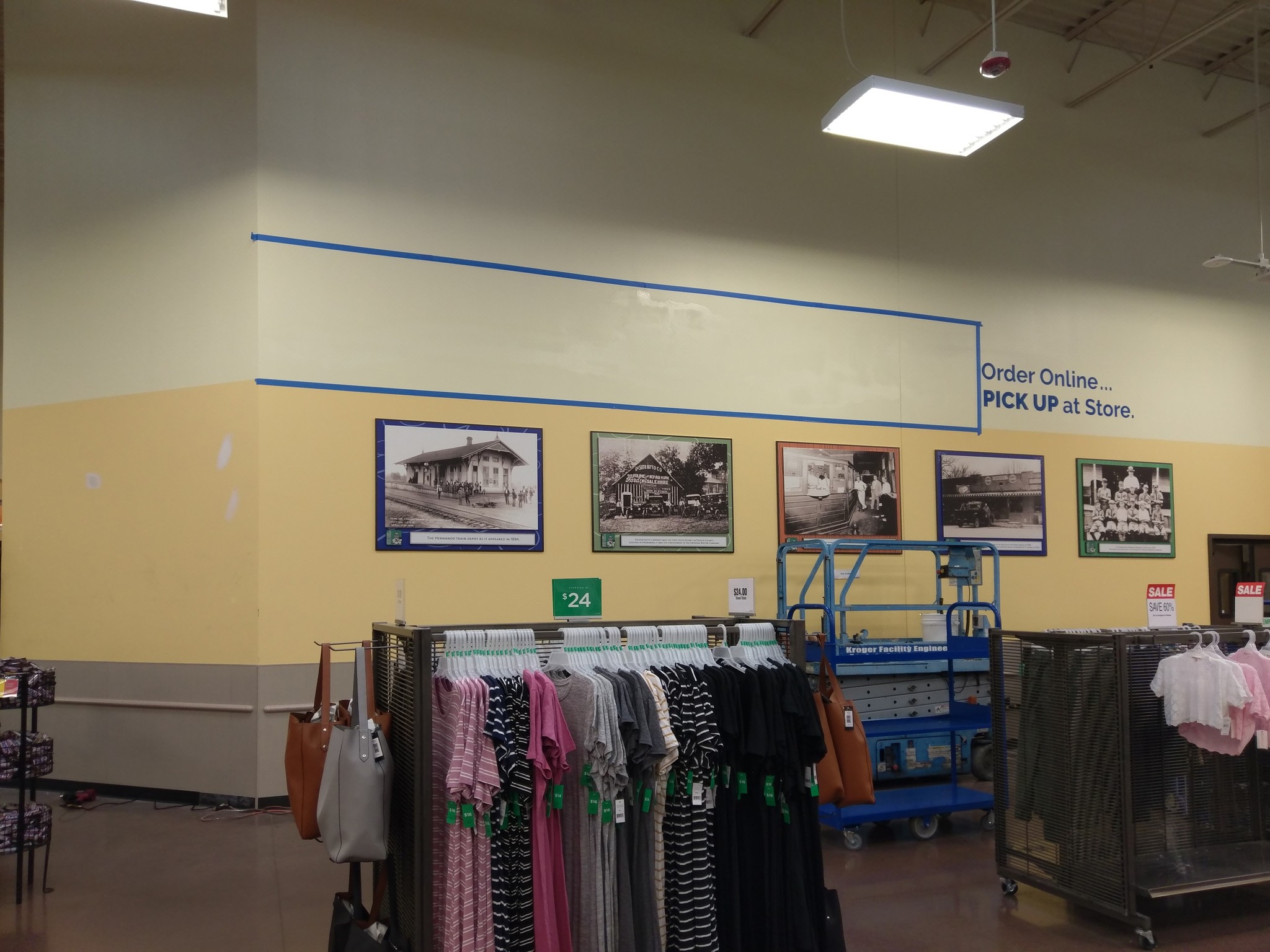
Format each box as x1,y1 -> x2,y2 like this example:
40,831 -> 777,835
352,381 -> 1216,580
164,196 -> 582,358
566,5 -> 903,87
787,601 -> 1001,851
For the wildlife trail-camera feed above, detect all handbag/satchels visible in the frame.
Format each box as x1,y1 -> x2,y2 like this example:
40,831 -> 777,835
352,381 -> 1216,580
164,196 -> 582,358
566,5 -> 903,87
285,640 -> 398,862
811,631 -> 876,808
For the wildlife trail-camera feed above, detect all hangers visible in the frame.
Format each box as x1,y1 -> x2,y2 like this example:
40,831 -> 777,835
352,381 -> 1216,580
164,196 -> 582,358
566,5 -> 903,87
432,623 -> 797,684
1031,625 -> 1270,657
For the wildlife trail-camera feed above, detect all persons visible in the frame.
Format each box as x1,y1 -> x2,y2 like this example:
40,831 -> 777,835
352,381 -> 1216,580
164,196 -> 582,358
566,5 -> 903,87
869,475 -> 882,510
661,498 -> 672,516
407,476 -> 415,484
616,499 -> 626,517
504,486 -> 535,508
1089,465 -> 1166,542
854,476 -> 868,513
807,467 -> 830,491
678,497 -> 701,517
983,503 -> 991,518
881,476 -> 892,496
433,478 -> 486,507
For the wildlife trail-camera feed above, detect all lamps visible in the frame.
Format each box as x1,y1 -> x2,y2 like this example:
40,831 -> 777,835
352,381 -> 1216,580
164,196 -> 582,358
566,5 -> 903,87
821,75 -> 1025,157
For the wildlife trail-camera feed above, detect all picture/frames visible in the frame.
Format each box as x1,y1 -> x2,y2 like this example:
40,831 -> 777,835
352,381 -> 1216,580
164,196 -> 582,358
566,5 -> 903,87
590,431 -> 734,553
1076,458 -> 1175,558
776,441 -> 902,555
935,449 -> 1047,556
375,417 -> 543,552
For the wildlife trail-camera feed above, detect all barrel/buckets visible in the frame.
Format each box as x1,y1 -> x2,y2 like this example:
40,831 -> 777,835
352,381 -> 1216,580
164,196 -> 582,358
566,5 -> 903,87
920,613 -> 960,641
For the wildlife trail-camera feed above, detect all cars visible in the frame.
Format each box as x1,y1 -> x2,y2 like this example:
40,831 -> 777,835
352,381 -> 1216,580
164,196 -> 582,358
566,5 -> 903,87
696,492 -> 728,521
599,495 -> 665,521
682,494 -> 702,518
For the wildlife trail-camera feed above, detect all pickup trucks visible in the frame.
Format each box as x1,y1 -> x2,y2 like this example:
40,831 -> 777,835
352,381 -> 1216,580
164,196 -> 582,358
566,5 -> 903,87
954,501 -> 994,528
